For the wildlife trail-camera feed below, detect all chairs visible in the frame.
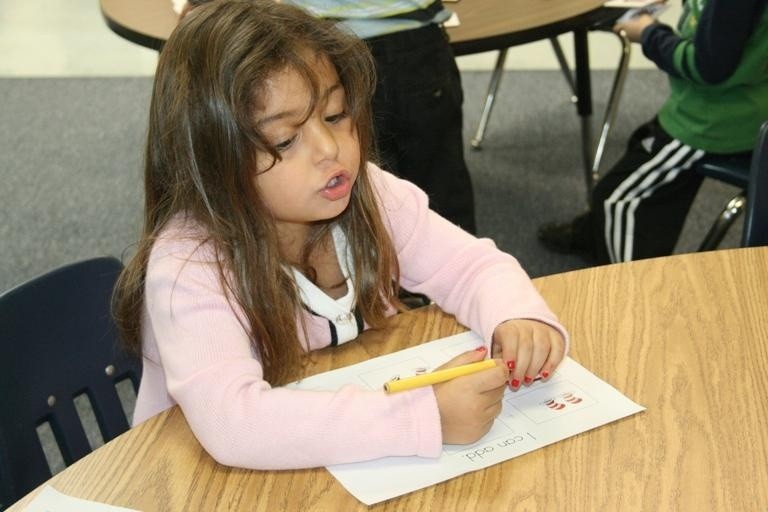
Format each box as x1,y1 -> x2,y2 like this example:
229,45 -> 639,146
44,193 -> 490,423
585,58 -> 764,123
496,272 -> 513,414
1,254 -> 146,512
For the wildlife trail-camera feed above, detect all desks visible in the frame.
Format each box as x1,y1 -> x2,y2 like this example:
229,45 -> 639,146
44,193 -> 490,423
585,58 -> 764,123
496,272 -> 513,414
0,244 -> 766,510
96,1 -> 635,215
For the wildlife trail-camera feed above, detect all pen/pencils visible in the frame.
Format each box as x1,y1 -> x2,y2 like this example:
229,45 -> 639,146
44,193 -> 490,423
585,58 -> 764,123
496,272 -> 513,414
384,359 -> 505,394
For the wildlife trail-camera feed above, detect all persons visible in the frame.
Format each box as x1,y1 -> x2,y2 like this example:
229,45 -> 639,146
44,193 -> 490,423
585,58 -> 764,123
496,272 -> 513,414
178,0 -> 480,309
532,0 -> 768,267
107,1 -> 572,471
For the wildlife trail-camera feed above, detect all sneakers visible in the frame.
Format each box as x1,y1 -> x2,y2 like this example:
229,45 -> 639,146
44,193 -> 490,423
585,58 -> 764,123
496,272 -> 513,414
536,211 -> 600,258
